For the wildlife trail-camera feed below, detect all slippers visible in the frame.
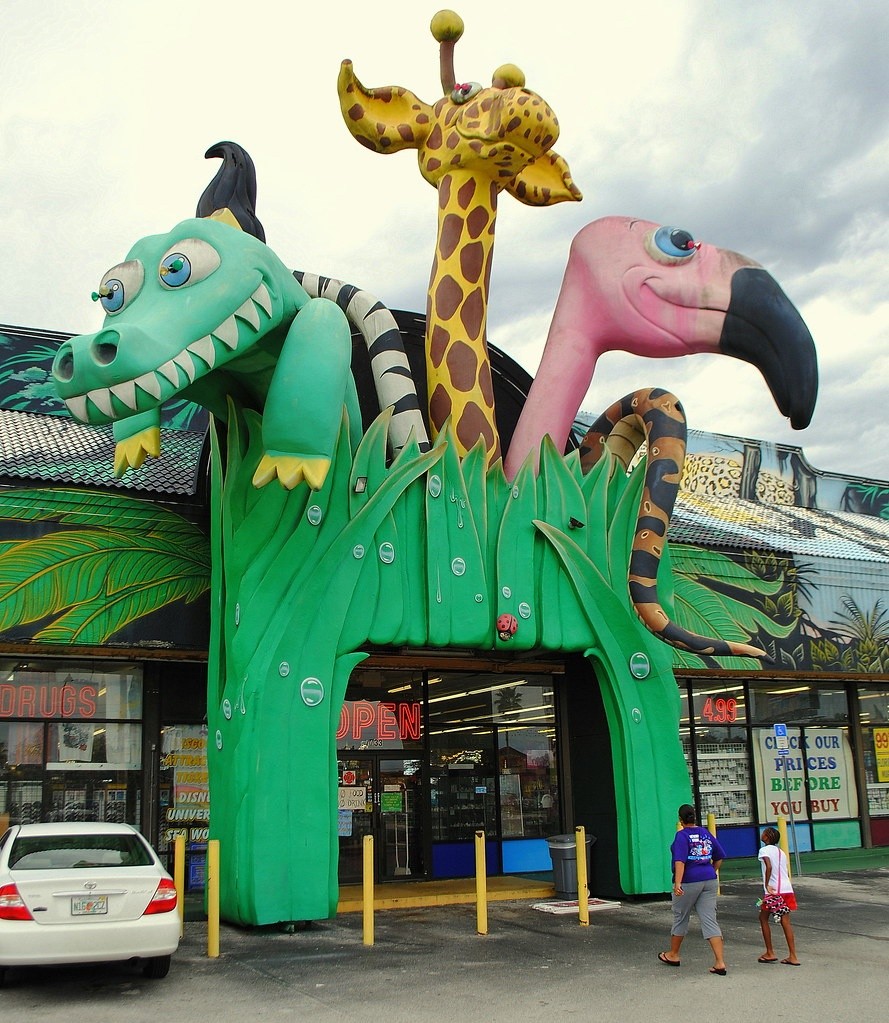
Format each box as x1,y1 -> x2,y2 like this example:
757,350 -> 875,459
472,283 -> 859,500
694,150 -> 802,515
758,956 -> 778,962
710,966 -> 726,975
658,952 -> 681,966
781,959 -> 801,966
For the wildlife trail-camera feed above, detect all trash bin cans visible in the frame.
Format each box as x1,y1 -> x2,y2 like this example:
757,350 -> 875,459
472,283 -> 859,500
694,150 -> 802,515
544,834 -> 598,901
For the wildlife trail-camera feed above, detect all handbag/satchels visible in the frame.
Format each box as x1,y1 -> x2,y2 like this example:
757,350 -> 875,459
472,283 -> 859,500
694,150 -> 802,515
763,893 -> 791,923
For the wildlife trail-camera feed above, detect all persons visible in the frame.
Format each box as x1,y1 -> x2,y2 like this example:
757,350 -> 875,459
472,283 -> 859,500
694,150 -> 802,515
758,827 -> 801,966
658,805 -> 727,976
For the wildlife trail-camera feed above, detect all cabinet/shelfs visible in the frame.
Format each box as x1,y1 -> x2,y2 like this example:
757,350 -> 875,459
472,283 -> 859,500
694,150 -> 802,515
431,805 -> 497,841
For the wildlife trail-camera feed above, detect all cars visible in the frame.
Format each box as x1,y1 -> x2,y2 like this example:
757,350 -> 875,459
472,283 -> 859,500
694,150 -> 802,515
1,820 -> 180,984
